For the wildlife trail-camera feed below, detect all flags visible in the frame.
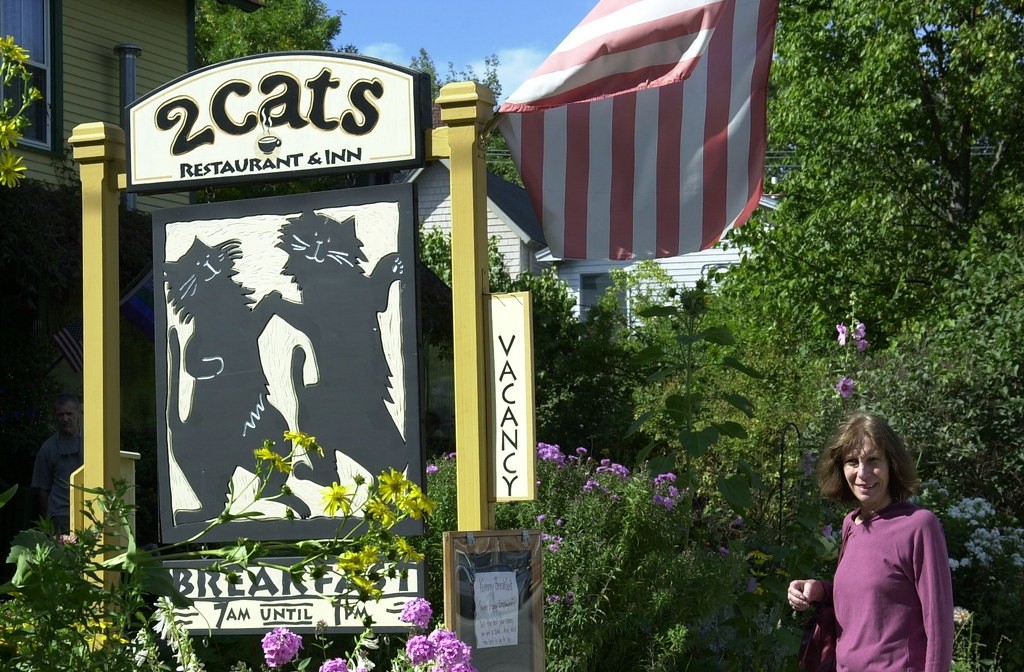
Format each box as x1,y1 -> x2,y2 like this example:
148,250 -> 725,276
118,269 -> 154,342
52,317 -> 83,375
492,0 -> 777,260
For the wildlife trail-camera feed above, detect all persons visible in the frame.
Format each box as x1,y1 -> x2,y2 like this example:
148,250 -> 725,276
30,391 -> 84,537
787,415 -> 953,672
